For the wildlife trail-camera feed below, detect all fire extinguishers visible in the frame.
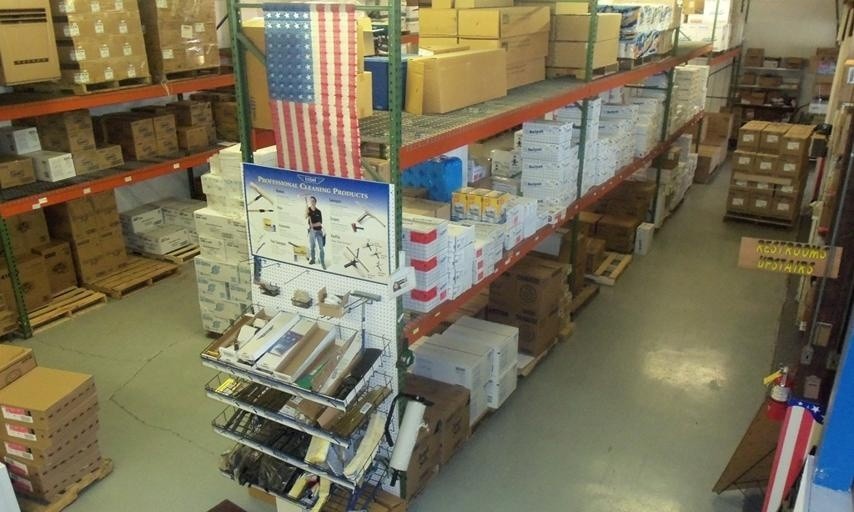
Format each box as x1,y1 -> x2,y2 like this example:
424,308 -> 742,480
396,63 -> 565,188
763,365 -> 792,421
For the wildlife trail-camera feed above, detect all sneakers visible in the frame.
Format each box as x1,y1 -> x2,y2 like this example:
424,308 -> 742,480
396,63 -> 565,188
309,260 -> 315,264
322,264 -> 326,269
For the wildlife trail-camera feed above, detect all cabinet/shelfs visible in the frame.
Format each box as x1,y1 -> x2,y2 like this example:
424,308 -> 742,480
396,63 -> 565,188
795,3 -> 853,407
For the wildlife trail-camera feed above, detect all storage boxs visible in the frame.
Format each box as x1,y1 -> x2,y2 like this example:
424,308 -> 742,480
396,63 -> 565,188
0,2 -> 269,335
1,344 -> 101,506
722,44 -> 838,227
192,3 -> 745,511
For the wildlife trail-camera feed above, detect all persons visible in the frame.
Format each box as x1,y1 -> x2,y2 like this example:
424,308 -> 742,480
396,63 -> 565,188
305,197 -> 327,270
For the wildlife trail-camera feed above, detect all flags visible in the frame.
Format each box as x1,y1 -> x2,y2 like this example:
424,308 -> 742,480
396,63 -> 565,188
262,3 -> 362,181
